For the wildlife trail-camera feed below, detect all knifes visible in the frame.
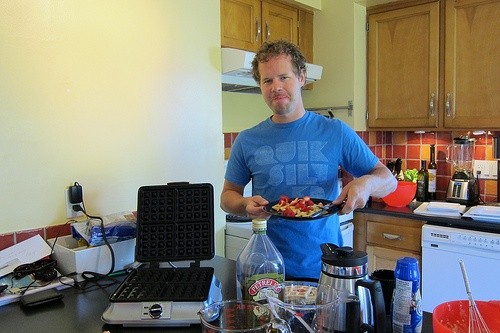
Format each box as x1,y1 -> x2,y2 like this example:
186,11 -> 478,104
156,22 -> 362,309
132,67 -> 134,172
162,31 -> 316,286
311,201 -> 336,218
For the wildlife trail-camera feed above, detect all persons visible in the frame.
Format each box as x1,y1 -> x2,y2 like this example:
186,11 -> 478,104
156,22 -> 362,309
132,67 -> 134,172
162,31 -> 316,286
221,41 -> 398,280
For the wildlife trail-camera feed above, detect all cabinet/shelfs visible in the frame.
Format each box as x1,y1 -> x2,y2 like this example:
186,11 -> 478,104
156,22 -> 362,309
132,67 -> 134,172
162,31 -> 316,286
354,211 -> 427,275
220,0 -> 299,53
366,0 -> 500,132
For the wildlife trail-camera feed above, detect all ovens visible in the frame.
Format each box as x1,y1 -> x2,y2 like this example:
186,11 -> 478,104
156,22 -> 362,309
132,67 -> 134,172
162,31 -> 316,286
226,221 -> 355,264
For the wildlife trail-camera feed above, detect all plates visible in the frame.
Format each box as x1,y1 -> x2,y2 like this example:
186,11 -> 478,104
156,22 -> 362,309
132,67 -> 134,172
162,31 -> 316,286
262,198 -> 340,220
413,201 -> 466,219
461,205 -> 500,223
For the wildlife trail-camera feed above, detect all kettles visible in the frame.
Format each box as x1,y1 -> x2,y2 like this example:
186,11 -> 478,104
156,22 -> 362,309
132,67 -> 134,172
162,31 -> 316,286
319,241 -> 386,333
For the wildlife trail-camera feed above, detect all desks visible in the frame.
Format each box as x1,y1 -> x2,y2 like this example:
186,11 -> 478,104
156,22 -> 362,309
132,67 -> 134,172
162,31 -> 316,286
0,255 -> 434,333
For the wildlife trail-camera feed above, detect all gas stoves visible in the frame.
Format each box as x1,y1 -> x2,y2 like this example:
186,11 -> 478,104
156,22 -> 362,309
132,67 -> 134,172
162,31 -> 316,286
221,46 -> 323,94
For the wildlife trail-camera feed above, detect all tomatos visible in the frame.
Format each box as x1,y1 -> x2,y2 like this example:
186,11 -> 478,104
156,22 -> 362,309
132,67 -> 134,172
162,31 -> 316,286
277,195 -> 315,216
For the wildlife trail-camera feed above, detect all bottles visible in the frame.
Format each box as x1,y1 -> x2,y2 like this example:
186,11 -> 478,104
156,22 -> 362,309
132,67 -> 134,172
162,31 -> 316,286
236,218 -> 285,303
427,144 -> 437,201
417,160 -> 428,202
390,256 -> 423,333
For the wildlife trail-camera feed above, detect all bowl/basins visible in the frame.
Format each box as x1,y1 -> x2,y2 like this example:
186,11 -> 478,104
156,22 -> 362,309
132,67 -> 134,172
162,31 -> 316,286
382,181 -> 417,208
432,300 -> 500,333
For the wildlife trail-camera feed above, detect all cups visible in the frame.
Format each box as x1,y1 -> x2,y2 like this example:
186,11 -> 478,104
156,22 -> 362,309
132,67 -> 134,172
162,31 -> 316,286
370,269 -> 396,316
197,299 -> 292,333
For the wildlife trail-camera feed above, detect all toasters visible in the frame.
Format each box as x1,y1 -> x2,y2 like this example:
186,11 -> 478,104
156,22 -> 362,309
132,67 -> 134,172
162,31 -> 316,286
447,171 -> 480,208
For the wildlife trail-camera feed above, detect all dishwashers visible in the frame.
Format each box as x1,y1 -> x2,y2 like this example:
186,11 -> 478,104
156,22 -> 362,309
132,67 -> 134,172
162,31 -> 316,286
421,221 -> 500,318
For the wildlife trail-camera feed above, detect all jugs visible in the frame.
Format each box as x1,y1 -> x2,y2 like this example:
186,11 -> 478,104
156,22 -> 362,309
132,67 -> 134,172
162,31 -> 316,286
265,282 -> 341,333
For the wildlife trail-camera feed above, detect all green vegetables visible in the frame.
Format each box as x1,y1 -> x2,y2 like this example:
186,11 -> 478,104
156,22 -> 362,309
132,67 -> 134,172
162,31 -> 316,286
403,168 -> 419,183
